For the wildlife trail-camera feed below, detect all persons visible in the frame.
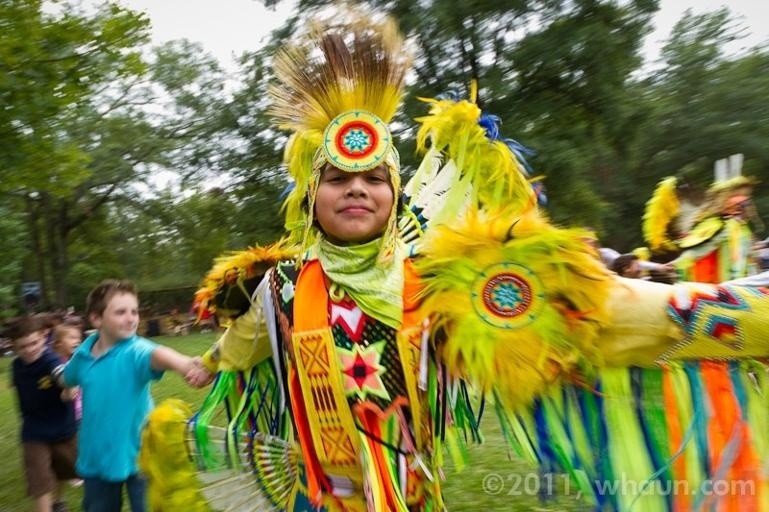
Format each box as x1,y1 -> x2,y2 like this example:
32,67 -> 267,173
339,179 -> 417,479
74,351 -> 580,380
0,163 -> 769,511
